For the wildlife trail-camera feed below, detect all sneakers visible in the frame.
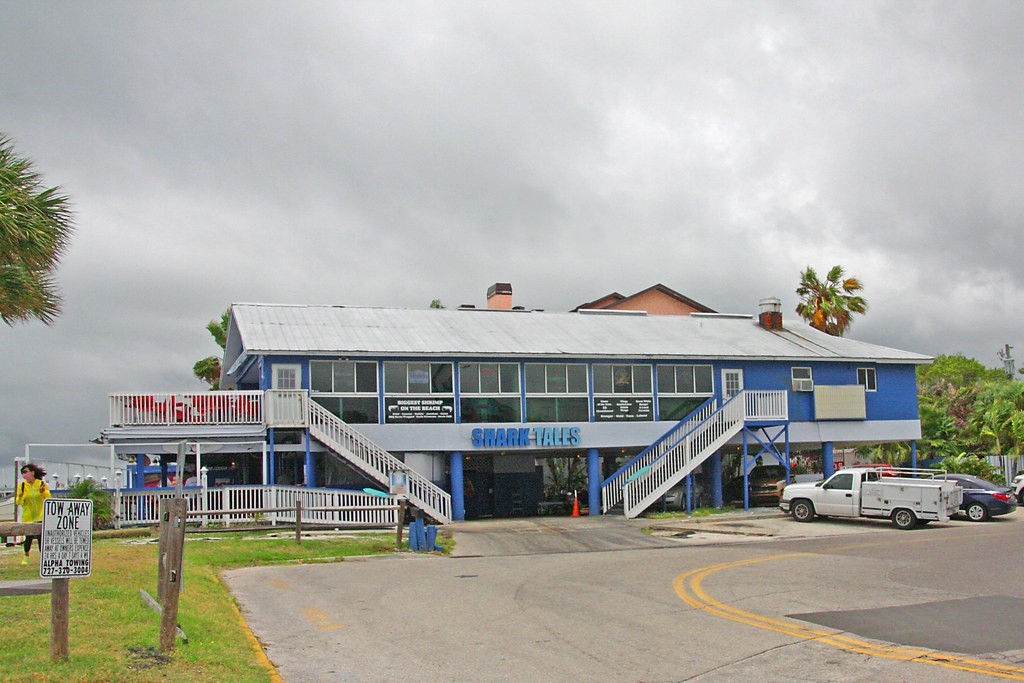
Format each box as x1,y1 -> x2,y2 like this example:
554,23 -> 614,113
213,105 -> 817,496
21,555 -> 31,566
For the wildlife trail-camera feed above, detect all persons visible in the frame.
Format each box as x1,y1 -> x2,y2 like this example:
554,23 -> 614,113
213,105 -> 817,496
14,461 -> 52,565
184,470 -> 203,518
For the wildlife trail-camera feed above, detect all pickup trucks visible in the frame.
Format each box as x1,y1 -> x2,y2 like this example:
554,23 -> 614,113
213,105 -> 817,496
779,467 -> 963,530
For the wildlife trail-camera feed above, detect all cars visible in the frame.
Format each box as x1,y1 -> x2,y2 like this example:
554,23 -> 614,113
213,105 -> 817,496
722,474 -> 749,503
1010,474 -> 1024,505
745,465 -> 797,505
927,473 -> 1017,522
651,478 -> 705,511
852,464 -> 901,477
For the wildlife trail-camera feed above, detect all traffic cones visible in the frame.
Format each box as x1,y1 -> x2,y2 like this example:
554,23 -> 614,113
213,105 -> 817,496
570,496 -> 580,517
